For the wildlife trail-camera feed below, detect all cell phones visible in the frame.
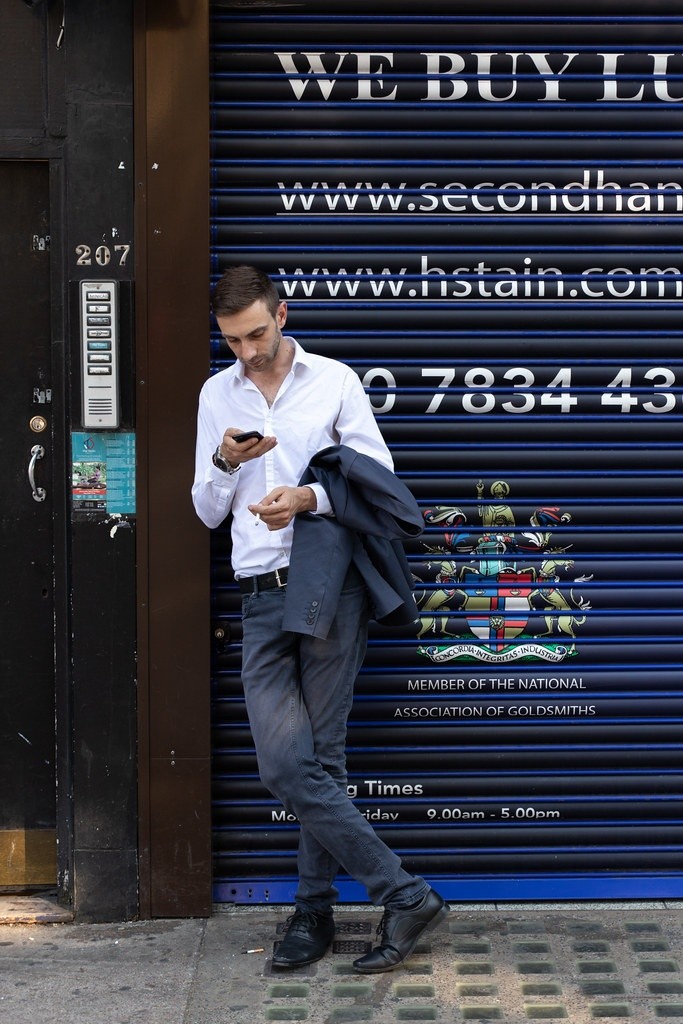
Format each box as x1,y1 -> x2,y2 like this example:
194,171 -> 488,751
232,431 -> 263,444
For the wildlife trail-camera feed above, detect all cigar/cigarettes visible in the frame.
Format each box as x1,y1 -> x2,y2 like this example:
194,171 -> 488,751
255,513 -> 260,526
247,949 -> 264,953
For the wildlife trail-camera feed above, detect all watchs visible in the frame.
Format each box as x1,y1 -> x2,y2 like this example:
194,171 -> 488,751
214,443 -> 241,474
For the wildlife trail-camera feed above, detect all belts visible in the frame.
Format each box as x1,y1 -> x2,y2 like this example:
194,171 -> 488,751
238,566 -> 289,594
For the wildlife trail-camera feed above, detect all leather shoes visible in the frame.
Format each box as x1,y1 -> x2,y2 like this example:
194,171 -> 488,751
273,906 -> 335,968
353,888 -> 450,973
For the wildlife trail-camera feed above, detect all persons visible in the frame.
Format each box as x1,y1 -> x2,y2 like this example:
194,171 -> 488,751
191,268 -> 450,973
87,466 -> 100,483
